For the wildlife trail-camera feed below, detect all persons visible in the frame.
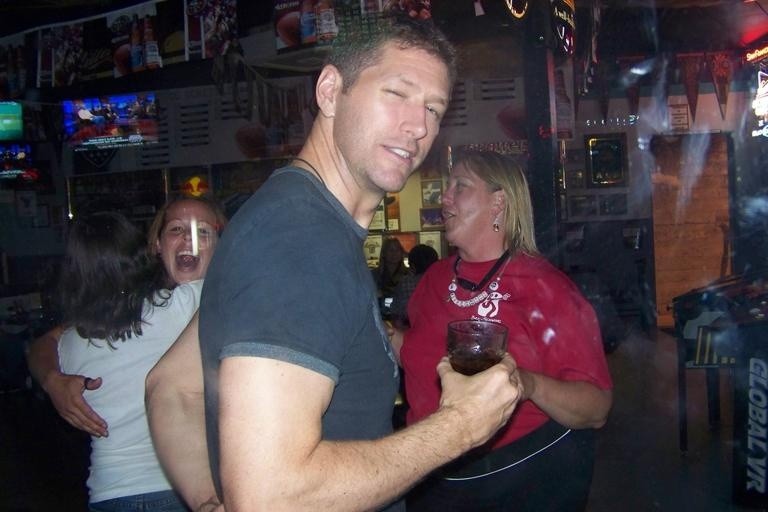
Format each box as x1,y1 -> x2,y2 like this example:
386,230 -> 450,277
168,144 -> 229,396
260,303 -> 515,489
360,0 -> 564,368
390,245 -> 441,326
371,237 -> 409,298
56,206 -> 205,512
72,92 -> 157,125
384,151 -> 614,511
27,193 -> 230,511
143,11 -> 526,511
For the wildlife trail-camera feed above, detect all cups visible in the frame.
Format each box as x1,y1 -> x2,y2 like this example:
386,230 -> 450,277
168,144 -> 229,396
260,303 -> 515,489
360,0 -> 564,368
444,316 -> 510,378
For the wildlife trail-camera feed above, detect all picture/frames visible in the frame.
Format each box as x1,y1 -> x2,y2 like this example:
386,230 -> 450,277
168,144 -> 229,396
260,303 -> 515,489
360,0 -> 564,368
564,131 -> 630,217
361,199 -> 383,269
419,152 -> 446,261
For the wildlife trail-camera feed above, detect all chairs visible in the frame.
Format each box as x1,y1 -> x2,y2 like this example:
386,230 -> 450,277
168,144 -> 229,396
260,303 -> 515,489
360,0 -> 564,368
670,275 -> 767,451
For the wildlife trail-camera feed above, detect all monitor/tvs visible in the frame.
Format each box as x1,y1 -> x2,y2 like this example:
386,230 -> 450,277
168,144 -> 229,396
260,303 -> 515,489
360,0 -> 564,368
0,98 -> 26,144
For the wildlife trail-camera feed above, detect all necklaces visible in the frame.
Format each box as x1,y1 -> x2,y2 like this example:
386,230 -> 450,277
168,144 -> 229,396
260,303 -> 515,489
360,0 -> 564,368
293,158 -> 326,189
453,248 -> 510,292
448,256 -> 514,307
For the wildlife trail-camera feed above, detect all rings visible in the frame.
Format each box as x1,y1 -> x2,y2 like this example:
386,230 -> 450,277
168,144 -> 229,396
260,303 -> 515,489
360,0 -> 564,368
83,376 -> 92,389
